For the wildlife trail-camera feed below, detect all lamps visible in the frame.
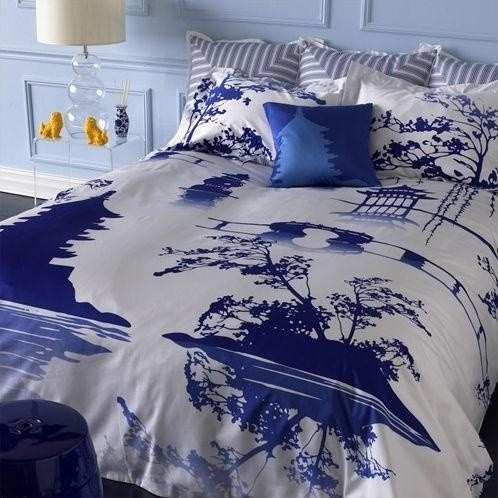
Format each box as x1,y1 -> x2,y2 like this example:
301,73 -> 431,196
35,0 -> 126,140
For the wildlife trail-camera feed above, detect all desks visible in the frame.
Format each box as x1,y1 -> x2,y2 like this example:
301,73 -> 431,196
32,134 -> 145,209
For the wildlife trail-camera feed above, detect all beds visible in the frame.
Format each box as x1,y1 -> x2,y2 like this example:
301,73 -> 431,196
0,151 -> 498,498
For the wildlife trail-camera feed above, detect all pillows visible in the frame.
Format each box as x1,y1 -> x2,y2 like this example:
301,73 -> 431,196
262,102 -> 382,188
183,28 -> 324,81
415,40 -> 495,91
141,63 -> 349,161
340,59 -> 496,188
292,32 -> 441,89
